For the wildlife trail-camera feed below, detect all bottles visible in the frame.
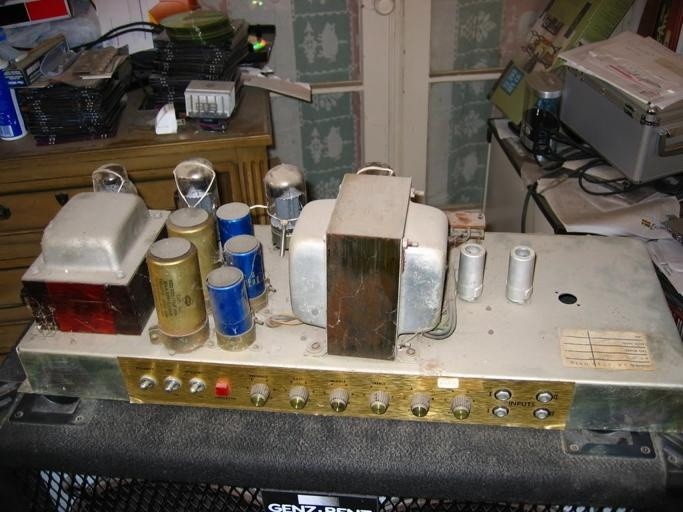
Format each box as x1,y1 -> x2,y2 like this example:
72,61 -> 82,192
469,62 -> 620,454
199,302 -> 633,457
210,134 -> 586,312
520,70 -> 564,154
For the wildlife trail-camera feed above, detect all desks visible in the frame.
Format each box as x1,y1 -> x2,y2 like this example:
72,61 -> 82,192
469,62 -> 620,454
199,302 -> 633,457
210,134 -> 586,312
0,85 -> 683,512
